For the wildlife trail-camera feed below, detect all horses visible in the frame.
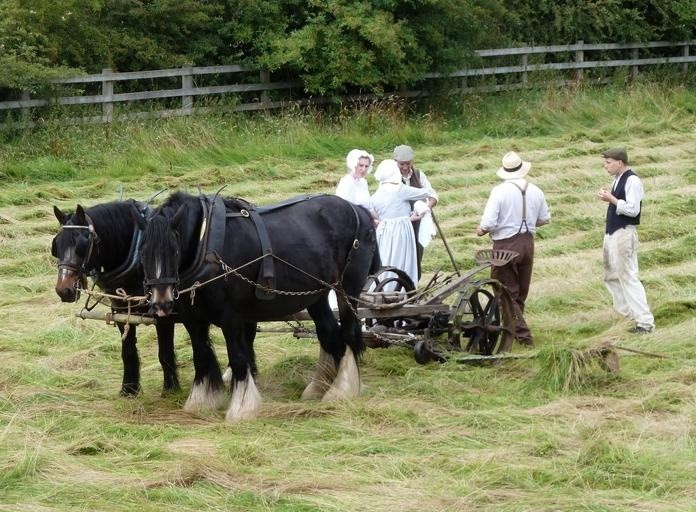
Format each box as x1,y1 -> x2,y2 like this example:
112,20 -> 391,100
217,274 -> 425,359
47,189 -> 259,396
127,188 -> 379,424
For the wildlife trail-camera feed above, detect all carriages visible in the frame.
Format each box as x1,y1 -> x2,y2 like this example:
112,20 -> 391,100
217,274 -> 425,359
47,182 -> 622,421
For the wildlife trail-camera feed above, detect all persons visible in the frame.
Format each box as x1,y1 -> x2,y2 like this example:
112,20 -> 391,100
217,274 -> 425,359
479,152 -> 550,345
598,147 -> 654,333
329,145 -> 433,331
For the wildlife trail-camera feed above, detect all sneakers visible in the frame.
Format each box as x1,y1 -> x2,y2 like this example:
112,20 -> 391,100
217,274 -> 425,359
628,327 -> 651,334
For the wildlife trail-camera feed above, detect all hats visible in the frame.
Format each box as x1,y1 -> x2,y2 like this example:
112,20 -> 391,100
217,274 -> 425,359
601,147 -> 627,163
496,151 -> 532,179
392,144 -> 414,162
346,148 -> 374,178
373,159 -> 403,185
